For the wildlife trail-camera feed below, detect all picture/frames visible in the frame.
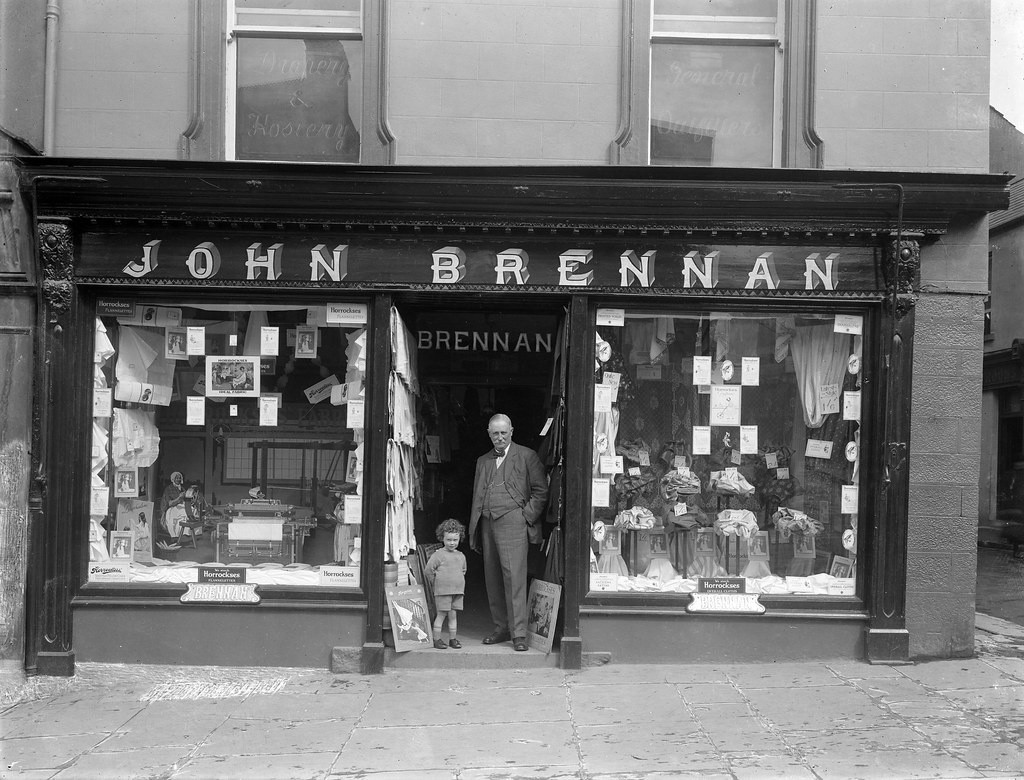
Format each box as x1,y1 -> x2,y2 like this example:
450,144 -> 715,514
385,585 -> 434,653
109,530 -> 135,562
829,555 -> 853,578
295,325 -> 318,358
114,467 -> 139,497
694,527 -> 717,556
647,531 -> 671,558
164,326 -> 189,360
598,525 -> 622,554
205,356 -> 260,397
747,531 -> 769,561
525,578 -> 562,654
794,533 -> 816,559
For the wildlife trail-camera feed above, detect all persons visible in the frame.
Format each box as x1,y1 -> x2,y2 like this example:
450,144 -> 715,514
468,413 -> 547,652
424,518 -> 467,650
397,621 -> 430,643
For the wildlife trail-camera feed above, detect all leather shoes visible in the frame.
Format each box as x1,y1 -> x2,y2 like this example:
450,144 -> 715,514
483,632 -> 511,644
513,637 -> 528,651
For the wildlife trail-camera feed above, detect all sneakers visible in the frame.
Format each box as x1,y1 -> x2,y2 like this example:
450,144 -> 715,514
449,639 -> 461,648
435,639 -> 447,649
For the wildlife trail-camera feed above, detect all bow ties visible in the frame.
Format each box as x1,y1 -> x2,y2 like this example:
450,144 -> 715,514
494,451 -> 505,459
